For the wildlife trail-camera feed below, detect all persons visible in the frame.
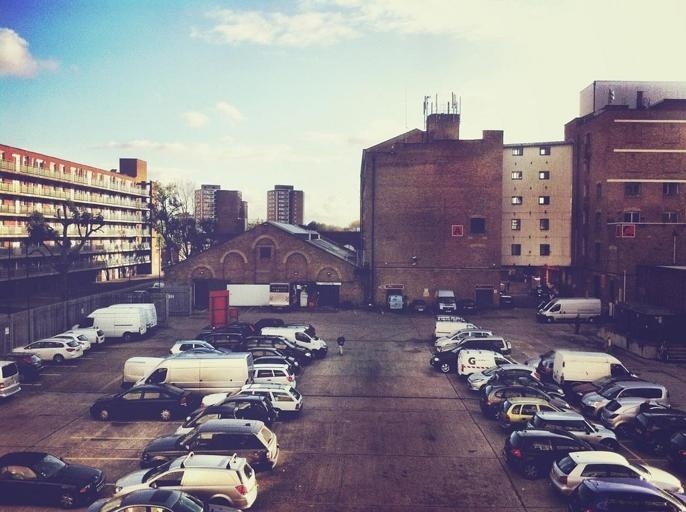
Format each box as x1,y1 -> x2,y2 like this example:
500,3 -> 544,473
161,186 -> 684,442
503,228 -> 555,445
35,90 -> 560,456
337,333 -> 345,356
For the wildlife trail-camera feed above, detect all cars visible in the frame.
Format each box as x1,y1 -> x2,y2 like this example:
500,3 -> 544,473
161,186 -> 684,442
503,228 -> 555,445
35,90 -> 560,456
129,290 -> 148,298
501,295 -> 513,308
412,299 -> 426,312
154,282 -> 164,288
0,327 -> 104,398
0,450 -> 106,508
459,299 -> 478,313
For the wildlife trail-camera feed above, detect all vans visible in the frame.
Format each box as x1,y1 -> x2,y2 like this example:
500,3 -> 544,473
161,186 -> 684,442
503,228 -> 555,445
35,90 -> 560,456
387,295 -> 403,311
434,289 -> 457,314
87,302 -> 159,341
536,296 -> 601,324
